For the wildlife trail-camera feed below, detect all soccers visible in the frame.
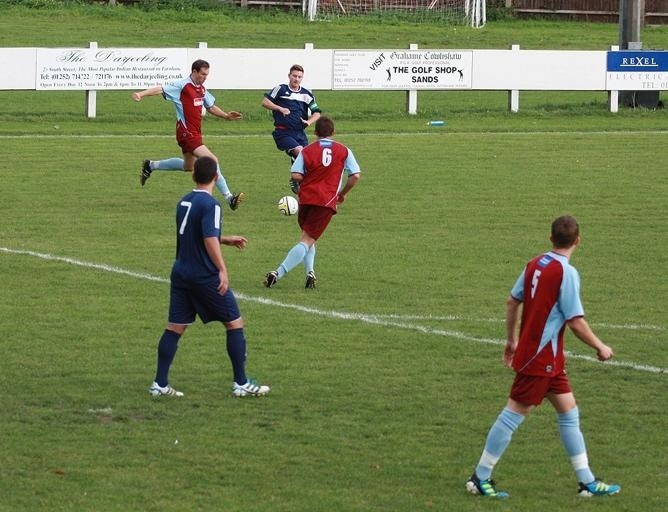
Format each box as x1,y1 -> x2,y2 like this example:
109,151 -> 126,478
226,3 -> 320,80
278,195 -> 298,216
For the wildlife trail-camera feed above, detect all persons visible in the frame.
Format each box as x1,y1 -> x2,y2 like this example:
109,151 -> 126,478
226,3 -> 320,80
263,65 -> 322,194
150,155 -> 271,398
265,117 -> 362,289
132,60 -> 247,211
468,216 -> 622,499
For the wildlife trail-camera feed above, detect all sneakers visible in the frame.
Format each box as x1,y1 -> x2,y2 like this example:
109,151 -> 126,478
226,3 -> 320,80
232,378 -> 271,397
139,159 -> 153,186
149,380 -> 185,399
289,178 -> 300,194
577,478 -> 622,498
465,471 -> 510,501
263,271 -> 278,288
229,190 -> 247,211
305,271 -> 316,289
